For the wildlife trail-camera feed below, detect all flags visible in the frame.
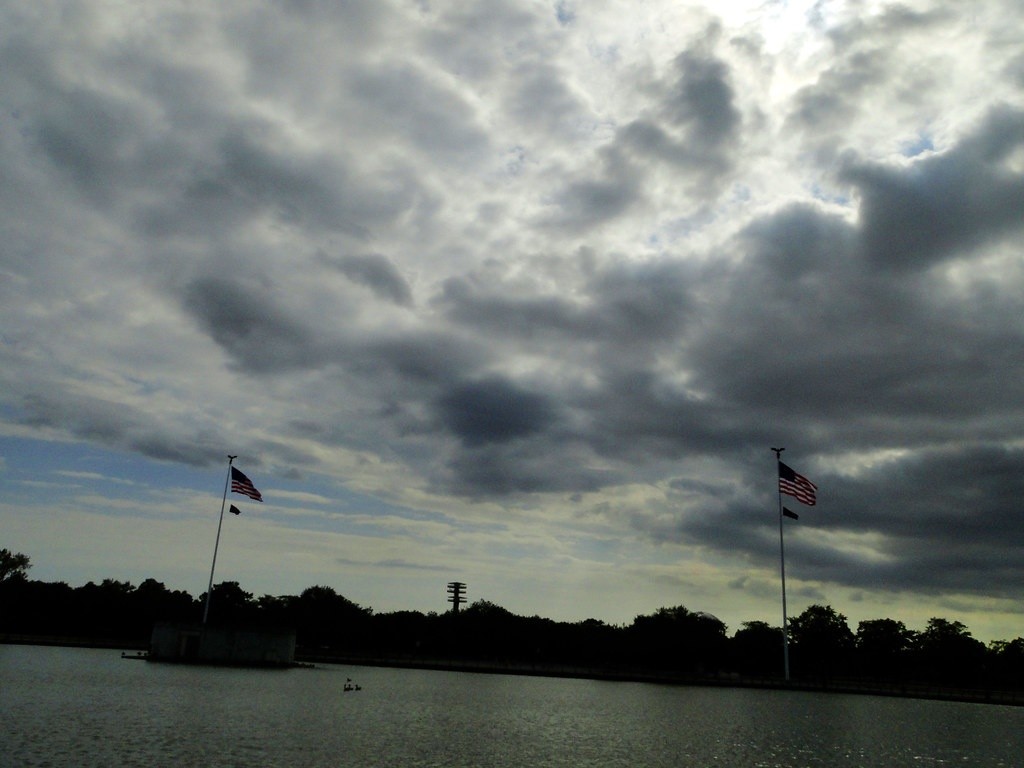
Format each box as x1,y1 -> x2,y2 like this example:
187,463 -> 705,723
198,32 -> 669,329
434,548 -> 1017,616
229,504 -> 241,515
778,459 -> 817,506
229,465 -> 264,502
783,507 -> 799,521
